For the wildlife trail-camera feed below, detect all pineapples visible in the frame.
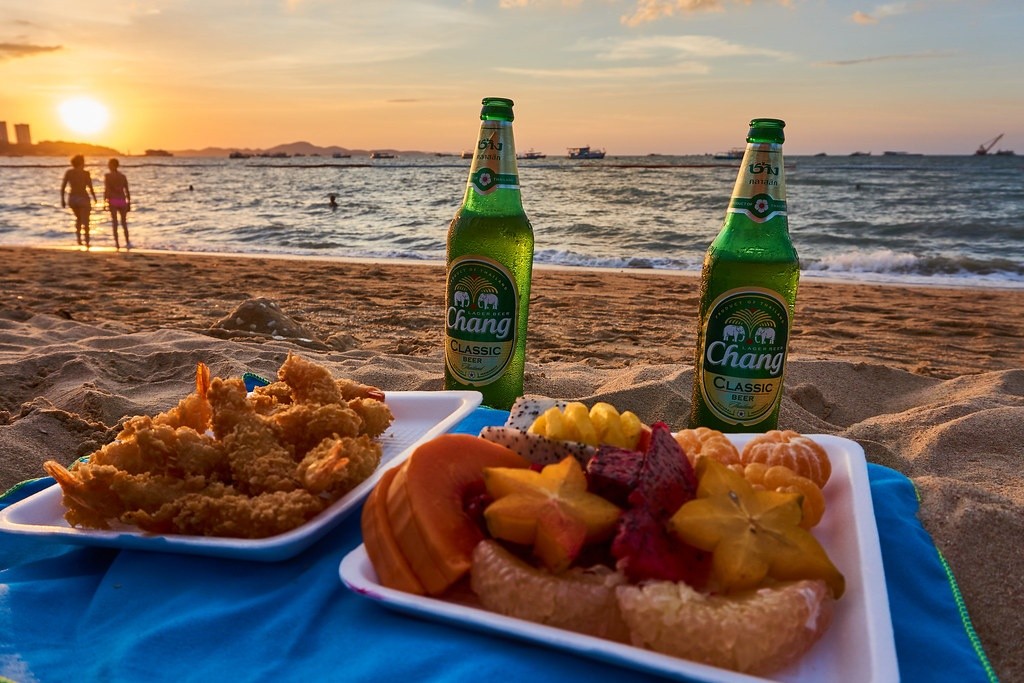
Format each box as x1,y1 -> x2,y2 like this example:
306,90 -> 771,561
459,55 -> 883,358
524,402 -> 643,451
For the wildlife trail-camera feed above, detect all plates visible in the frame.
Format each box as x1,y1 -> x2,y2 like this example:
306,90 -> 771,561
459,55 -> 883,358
0,389 -> 484,567
339,433 -> 901,683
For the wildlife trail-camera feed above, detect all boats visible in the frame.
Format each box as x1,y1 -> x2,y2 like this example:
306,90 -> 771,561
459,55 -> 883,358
712,148 -> 746,161
515,151 -> 546,162
461,151 -> 473,159
332,153 -> 351,158
369,152 -> 395,160
567,145 -> 607,161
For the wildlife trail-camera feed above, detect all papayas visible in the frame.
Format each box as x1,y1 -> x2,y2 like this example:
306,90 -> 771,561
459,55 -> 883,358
362,433 -> 538,595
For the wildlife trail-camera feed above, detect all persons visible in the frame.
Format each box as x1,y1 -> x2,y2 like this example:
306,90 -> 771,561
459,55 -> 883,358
328,196 -> 337,207
61,155 -> 96,247
104,159 -> 133,249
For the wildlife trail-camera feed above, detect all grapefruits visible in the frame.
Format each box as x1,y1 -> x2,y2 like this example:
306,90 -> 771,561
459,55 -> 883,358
469,540 -> 632,643
614,577 -> 836,673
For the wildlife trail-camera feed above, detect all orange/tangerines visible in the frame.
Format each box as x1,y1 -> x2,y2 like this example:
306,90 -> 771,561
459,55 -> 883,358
670,424 -> 741,472
729,463 -> 825,530
741,429 -> 834,487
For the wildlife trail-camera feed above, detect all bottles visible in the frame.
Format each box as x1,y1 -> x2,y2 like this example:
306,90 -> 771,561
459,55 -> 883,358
445,97 -> 535,413
687,117 -> 800,433
